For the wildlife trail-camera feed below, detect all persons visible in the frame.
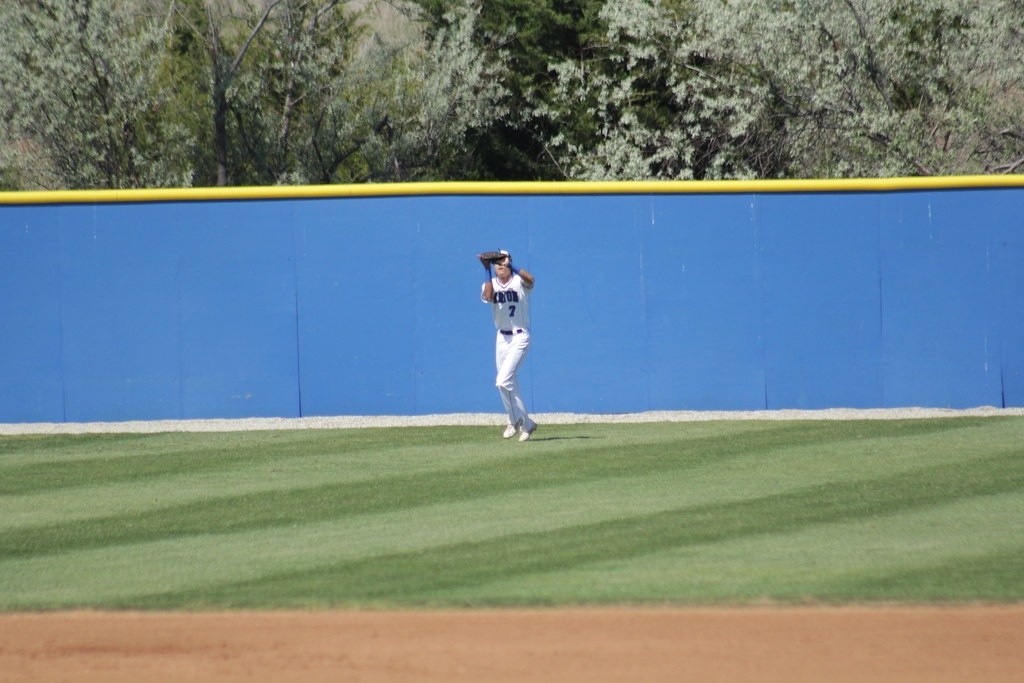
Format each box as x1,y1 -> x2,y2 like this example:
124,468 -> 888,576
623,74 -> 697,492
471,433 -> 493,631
481,249 -> 537,441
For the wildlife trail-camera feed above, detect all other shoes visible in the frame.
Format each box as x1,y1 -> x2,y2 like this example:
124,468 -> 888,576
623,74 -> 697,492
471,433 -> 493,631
503,417 -> 523,439
518,422 -> 537,441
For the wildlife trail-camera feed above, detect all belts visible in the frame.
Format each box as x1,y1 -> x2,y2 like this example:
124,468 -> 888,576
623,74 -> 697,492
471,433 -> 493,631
500,329 -> 522,335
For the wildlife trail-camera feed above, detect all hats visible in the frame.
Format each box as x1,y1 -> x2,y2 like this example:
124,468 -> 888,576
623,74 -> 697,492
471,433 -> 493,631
500,250 -> 512,263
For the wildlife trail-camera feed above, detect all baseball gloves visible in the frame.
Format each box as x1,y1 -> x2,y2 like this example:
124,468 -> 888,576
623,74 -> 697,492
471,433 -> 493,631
478,252 -> 507,264
